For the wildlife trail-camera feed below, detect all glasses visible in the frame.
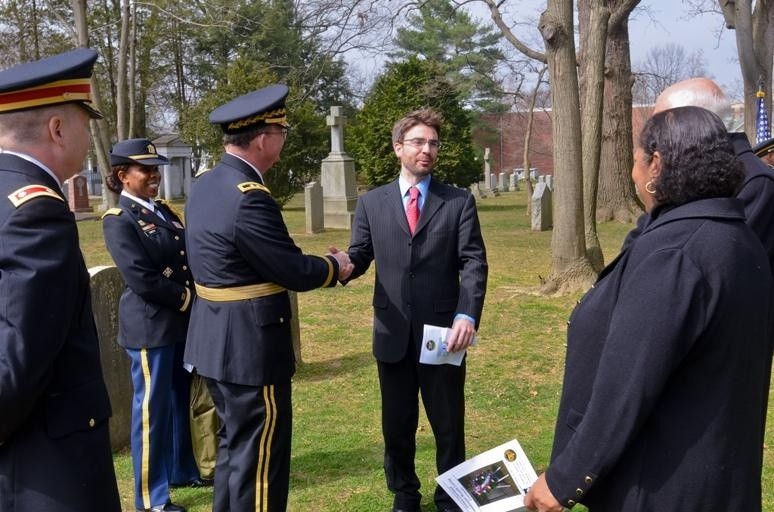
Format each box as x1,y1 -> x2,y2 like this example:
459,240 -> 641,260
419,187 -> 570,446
402,138 -> 440,147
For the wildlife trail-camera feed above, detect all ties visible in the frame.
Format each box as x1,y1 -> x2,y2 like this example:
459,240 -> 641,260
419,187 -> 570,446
407,188 -> 419,237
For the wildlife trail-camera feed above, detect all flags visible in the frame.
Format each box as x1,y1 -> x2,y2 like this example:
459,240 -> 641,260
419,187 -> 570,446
754,96 -> 771,143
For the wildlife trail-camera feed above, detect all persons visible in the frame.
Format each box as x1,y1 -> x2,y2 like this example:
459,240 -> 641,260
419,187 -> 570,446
101,137 -> 214,512
1,46 -> 120,511
329,106 -> 486,512
524,106 -> 774,512
652,77 -> 773,259
752,139 -> 774,170
180,84 -> 351,512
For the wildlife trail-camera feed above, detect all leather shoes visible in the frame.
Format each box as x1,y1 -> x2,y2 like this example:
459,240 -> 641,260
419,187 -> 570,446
135,502 -> 185,512
179,478 -> 214,486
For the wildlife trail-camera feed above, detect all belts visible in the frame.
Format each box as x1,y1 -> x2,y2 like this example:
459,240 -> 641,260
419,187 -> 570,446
194,283 -> 285,301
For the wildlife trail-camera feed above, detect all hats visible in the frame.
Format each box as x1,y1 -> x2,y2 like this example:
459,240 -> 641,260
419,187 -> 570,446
0,49 -> 104,119
209,84 -> 291,135
109,138 -> 168,165
